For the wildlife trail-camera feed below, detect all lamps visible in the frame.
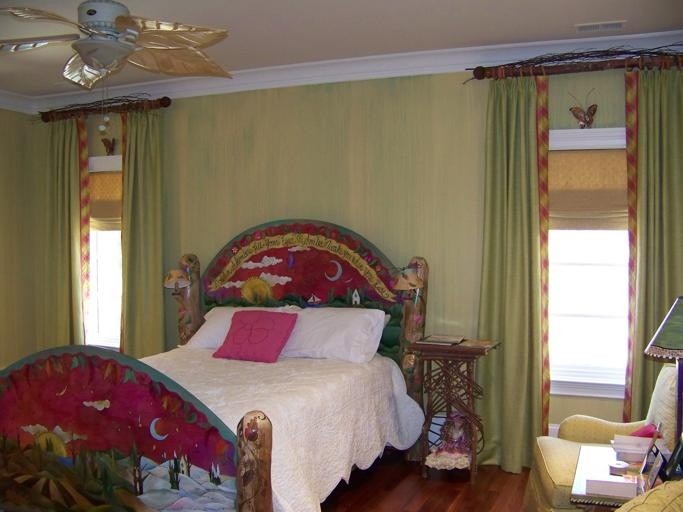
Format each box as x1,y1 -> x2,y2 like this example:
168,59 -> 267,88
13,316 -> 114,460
179,307 -> 288,357
393,269 -> 425,305
71,39 -> 135,73
164,269 -> 191,296
643,296 -> 683,480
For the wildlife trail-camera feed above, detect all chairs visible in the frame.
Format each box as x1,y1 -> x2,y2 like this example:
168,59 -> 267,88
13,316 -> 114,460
522,361 -> 682,512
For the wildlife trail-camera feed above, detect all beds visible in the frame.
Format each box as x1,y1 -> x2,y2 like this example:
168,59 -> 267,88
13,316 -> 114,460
2,220 -> 428,512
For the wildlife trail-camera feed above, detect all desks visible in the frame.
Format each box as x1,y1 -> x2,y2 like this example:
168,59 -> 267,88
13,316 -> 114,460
571,443 -> 681,512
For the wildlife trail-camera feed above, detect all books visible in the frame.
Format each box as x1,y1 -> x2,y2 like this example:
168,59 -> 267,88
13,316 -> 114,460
610,434 -> 672,461
416,335 -> 453,346
585,474 -> 637,499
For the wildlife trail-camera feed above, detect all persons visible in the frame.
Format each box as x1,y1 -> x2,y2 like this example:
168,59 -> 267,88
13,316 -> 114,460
425,413 -> 472,469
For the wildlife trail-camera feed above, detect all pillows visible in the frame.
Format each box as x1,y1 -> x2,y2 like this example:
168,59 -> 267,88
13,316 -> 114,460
213,311 -> 298,363
289,308 -> 385,363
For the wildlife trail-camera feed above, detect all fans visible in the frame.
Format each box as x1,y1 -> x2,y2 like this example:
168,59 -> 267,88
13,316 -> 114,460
0,1 -> 235,90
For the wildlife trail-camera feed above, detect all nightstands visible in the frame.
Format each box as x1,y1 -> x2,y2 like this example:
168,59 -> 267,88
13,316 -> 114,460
409,334 -> 501,483
